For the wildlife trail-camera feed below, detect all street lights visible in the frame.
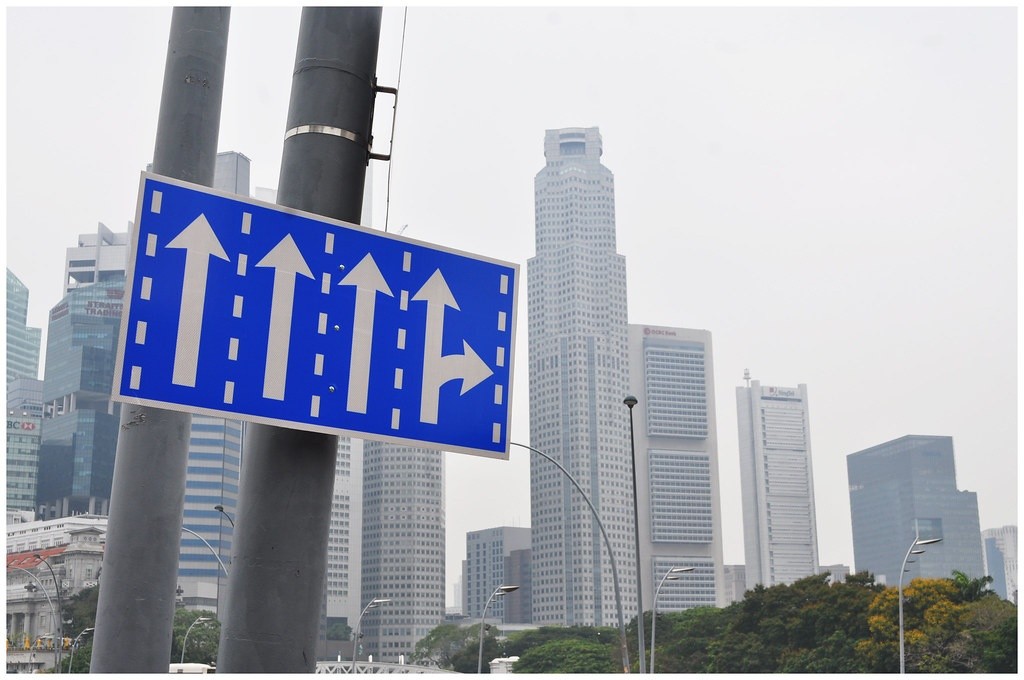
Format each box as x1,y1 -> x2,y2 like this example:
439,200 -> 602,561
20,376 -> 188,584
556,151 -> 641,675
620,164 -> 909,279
477,581 -> 520,674
352,596 -> 392,673
32,553 -> 64,673
179,615 -> 211,673
68,626 -> 96,673
650,565 -> 694,672
622,394 -> 650,673
896,536 -> 940,673
